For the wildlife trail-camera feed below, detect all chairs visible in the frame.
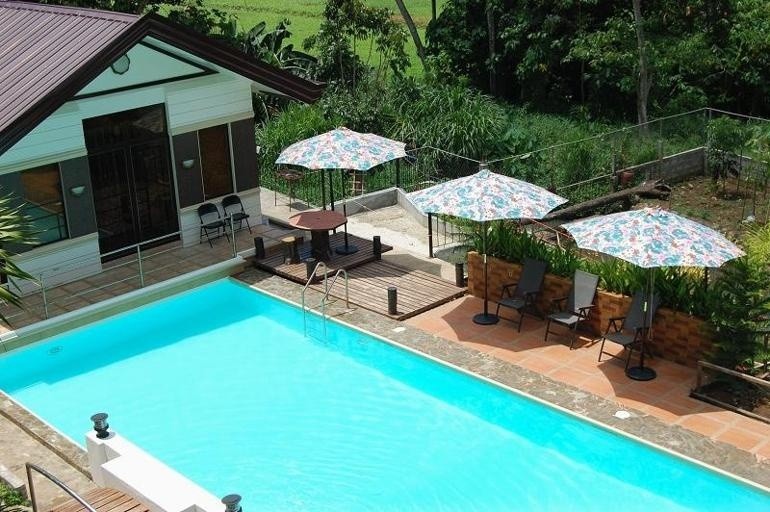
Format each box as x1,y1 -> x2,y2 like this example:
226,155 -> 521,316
197,195 -> 251,248
274,146 -> 308,211
496,255 -> 662,372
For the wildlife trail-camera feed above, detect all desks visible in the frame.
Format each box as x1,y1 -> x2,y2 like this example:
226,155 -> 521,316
287,210 -> 347,263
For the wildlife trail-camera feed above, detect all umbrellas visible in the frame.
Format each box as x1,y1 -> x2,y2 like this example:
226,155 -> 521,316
406,169 -> 570,315
560,207 -> 747,369
274,126 -> 408,248
